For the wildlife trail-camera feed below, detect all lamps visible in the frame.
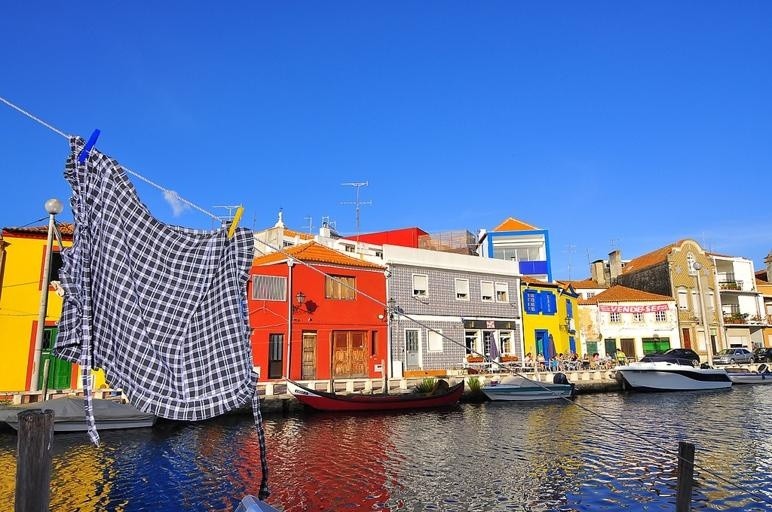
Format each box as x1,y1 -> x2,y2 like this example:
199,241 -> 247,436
291,291 -> 306,315
384,297 -> 397,318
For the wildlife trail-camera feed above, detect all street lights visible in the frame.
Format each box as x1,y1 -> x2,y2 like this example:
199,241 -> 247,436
30,199 -> 63,392
387,298 -> 396,377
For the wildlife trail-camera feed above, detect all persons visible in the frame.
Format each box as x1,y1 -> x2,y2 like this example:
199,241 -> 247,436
524,349 -> 628,371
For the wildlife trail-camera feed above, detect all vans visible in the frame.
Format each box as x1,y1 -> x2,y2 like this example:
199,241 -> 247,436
645,348 -> 700,366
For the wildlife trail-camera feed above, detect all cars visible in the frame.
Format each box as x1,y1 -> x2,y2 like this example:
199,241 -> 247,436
712,348 -> 772,365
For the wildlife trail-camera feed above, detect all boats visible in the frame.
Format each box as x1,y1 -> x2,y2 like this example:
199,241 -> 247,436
0,395 -> 158,432
615,357 -> 733,391
282,376 -> 464,411
728,364 -> 772,384
480,373 -> 575,400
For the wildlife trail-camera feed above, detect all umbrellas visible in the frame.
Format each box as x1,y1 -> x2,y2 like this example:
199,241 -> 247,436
549,334 -> 557,359
490,333 -> 499,360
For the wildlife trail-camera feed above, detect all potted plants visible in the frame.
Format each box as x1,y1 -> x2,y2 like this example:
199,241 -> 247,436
720,282 -> 743,290
724,312 -> 750,324
